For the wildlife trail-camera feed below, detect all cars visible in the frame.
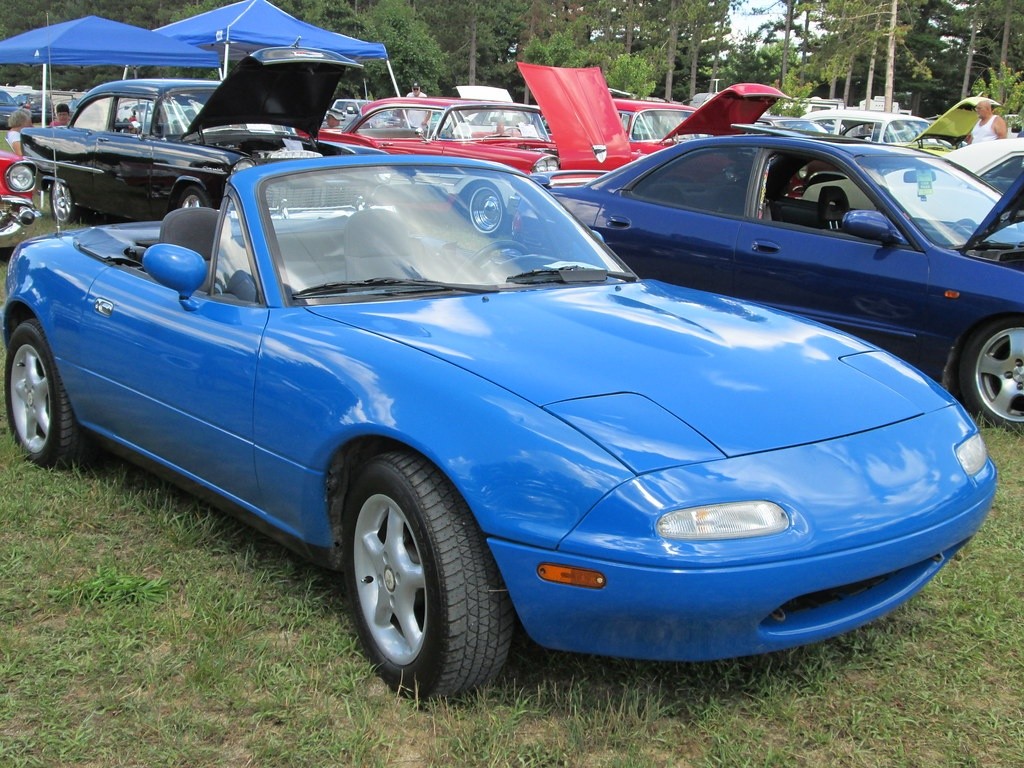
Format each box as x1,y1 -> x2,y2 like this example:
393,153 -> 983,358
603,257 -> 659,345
332,98 -> 404,130
0,152 -> 42,237
512,123 -> 1024,433
0,90 -> 19,130
13,93 -> 55,125
296,62 -> 641,236
610,84 -> 793,190
441,85 -> 542,139
20,35 -> 364,222
802,138 -> 1024,221
798,95 -> 1001,155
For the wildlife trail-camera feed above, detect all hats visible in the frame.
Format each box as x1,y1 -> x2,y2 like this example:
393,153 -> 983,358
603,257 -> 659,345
412,82 -> 420,87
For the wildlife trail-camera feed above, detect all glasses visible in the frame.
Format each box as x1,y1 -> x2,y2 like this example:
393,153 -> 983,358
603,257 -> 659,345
975,107 -> 983,111
413,88 -> 419,91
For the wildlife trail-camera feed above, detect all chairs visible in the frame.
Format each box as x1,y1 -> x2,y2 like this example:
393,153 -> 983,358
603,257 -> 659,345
817,185 -> 851,233
158,206 -> 238,293
343,208 -> 417,282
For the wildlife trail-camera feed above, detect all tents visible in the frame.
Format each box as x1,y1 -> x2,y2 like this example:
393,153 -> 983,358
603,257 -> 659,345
0,14 -> 222,130
117,0 -> 412,128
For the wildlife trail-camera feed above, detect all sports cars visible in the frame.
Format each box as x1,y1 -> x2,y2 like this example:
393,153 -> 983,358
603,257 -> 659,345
0,153 -> 998,713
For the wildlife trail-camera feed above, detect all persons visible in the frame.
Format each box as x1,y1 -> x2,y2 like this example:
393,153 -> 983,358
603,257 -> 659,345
320,107 -> 346,127
405,82 -> 432,138
7,107 -> 34,156
48,103 -> 71,127
966,101 -> 1007,144
